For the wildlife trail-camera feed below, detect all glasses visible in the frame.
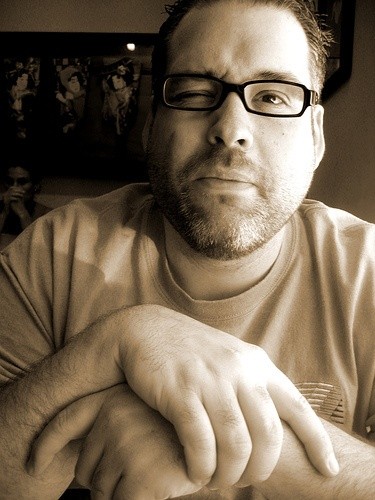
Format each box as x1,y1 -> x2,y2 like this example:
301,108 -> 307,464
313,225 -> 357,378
152,72 -> 319,118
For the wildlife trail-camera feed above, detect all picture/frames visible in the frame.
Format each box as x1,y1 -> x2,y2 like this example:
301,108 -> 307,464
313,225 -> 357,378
0,32 -> 159,182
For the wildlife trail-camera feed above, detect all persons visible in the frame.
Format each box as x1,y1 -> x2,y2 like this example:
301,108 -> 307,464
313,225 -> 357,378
1,152 -> 53,252
1,0 -> 375,500
